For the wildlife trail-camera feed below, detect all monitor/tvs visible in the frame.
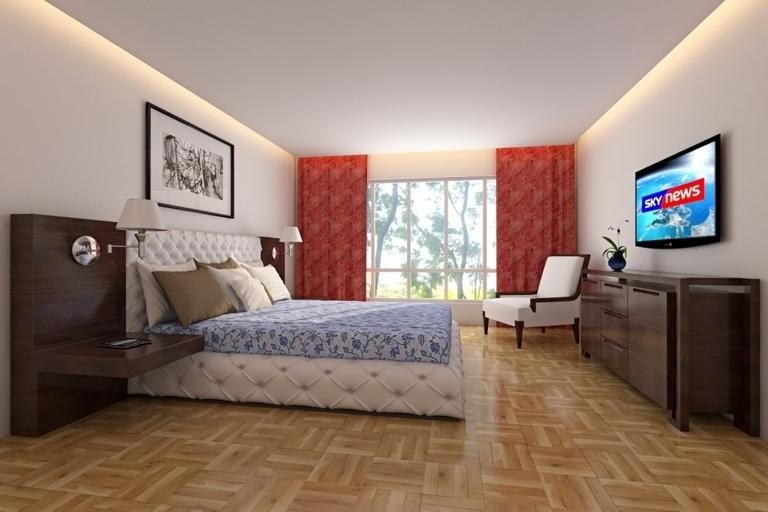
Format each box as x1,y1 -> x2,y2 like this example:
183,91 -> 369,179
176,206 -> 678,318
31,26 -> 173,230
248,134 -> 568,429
635,133 -> 722,249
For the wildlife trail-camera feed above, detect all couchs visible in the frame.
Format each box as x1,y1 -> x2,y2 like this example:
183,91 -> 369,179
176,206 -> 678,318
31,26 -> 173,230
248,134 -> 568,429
478,251 -> 589,351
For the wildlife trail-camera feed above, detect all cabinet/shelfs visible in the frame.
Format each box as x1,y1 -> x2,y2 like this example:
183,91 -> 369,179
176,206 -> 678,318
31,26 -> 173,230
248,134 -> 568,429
579,268 -> 762,439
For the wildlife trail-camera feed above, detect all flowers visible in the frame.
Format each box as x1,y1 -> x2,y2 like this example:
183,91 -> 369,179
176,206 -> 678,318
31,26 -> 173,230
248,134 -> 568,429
599,219 -> 630,261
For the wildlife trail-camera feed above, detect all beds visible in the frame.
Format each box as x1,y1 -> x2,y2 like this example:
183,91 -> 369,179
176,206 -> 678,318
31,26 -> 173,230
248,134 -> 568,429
122,227 -> 467,423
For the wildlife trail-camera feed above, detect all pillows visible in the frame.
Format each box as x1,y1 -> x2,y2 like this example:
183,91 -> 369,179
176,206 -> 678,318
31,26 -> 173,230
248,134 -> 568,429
134,252 -> 294,333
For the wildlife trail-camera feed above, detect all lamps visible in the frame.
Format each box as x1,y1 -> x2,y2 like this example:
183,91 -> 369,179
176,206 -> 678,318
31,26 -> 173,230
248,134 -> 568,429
67,198 -> 167,268
270,226 -> 304,261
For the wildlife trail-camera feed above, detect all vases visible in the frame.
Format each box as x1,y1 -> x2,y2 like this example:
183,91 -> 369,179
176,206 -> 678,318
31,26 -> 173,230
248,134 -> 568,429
607,254 -> 625,270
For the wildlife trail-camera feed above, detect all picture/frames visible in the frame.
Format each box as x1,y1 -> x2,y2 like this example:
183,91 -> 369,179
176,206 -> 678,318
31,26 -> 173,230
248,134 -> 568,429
142,99 -> 237,219
